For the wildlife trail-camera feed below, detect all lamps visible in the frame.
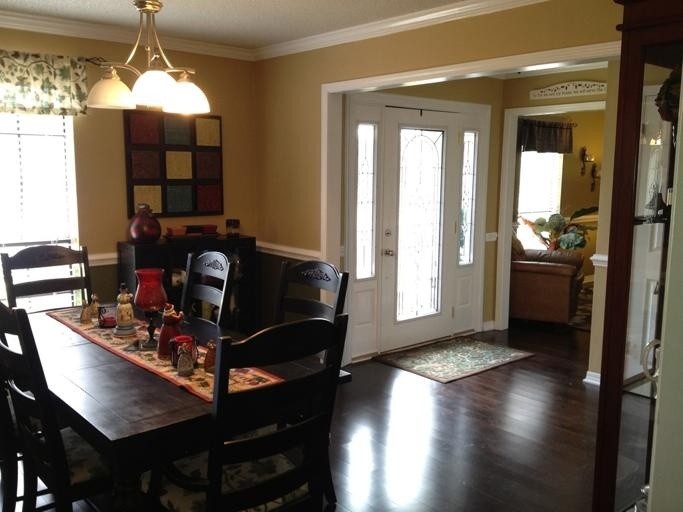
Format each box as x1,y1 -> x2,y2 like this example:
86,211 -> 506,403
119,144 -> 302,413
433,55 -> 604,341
84,0 -> 213,118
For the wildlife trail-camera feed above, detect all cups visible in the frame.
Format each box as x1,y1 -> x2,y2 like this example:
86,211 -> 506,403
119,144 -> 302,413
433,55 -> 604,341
98,305 -> 118,328
226,218 -> 240,238
170,337 -> 198,367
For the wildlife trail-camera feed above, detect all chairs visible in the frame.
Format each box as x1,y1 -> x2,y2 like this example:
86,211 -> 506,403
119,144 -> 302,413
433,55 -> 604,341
509,227 -> 584,323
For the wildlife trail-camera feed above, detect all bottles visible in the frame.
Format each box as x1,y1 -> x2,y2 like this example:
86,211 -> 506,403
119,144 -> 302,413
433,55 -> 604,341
177,339 -> 216,377
79,293 -> 100,324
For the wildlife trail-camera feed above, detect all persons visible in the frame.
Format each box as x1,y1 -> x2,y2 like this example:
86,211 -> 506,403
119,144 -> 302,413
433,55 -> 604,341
176,342 -> 194,377
115,281 -> 136,327
156,303 -> 184,359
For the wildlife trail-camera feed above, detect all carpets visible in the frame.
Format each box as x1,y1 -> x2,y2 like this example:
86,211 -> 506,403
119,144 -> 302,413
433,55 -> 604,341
372,335 -> 534,382
